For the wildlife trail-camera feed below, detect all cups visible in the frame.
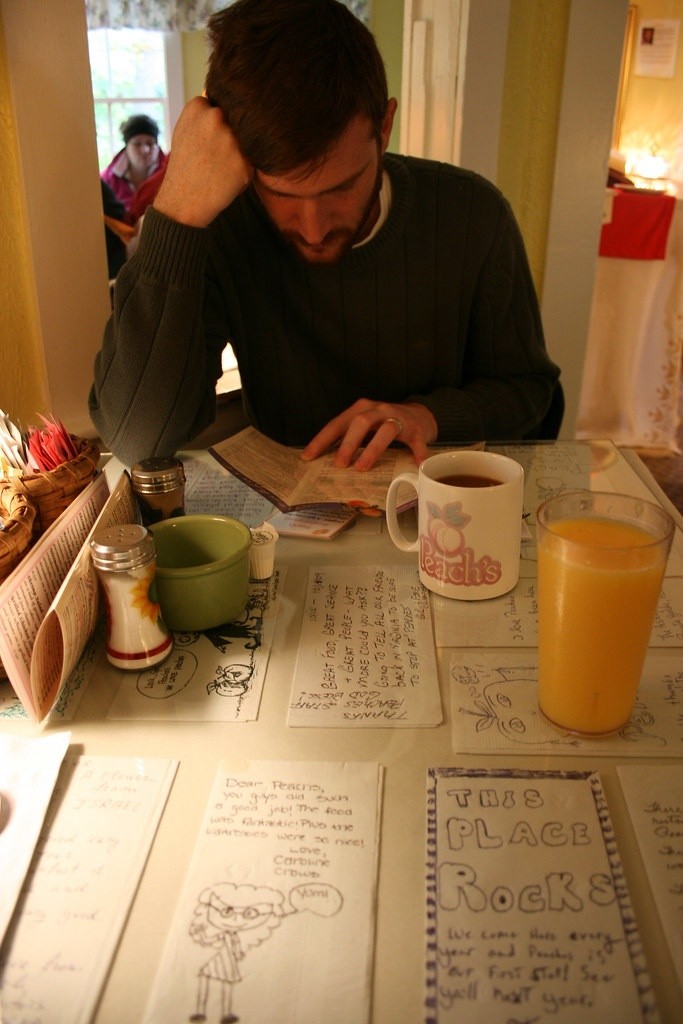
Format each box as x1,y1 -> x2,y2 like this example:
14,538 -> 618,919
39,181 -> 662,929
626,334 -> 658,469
386,451 -> 525,600
537,493 -> 675,738
250,524 -> 278,579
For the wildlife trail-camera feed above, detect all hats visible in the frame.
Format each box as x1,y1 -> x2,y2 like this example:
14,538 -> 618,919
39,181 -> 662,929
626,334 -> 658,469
121,113 -> 160,145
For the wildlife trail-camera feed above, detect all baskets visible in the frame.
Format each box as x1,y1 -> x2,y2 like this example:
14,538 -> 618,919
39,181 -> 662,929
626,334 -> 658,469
0,483 -> 37,582
0,427 -> 101,533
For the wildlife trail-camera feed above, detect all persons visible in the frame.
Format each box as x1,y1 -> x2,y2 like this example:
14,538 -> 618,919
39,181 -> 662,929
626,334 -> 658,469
100,114 -> 171,225
100,179 -> 137,279
87,0 -> 566,471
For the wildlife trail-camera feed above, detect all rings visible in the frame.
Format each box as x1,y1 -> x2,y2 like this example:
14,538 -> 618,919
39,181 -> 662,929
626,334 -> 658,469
388,418 -> 402,431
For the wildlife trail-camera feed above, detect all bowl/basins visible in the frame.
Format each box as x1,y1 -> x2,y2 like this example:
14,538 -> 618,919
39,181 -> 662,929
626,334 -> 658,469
147,516 -> 251,631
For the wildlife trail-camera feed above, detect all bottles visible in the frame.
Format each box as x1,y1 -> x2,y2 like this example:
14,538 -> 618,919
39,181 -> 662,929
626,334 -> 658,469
131,457 -> 186,527
90,524 -> 174,673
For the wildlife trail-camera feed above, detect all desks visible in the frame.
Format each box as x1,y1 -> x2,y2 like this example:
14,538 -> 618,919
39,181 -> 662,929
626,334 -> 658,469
577,186 -> 683,454
0,438 -> 683,1024
